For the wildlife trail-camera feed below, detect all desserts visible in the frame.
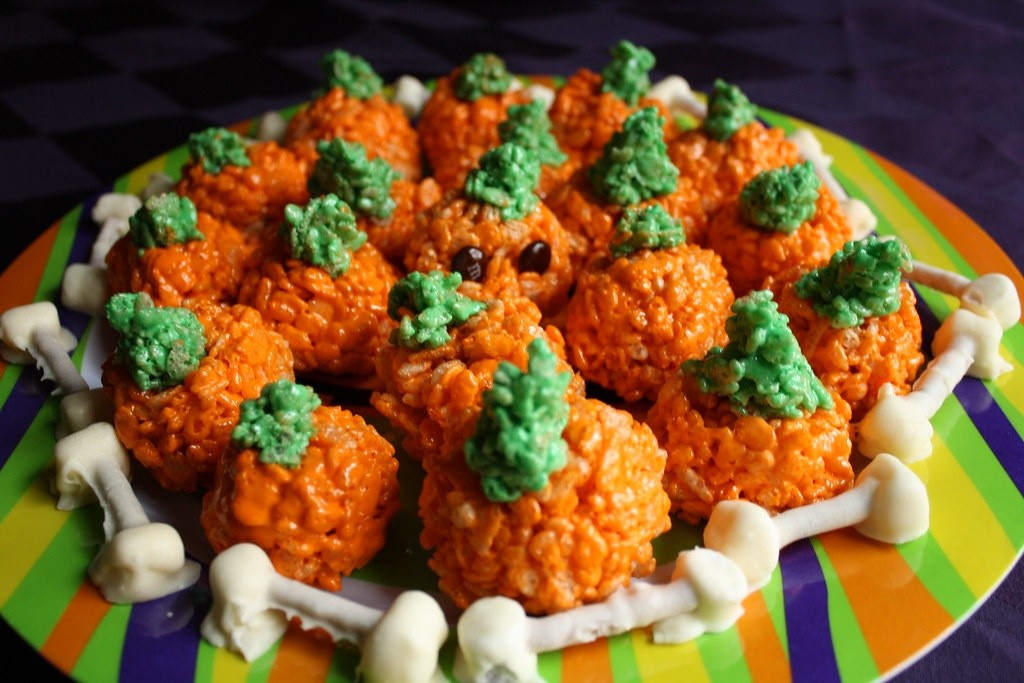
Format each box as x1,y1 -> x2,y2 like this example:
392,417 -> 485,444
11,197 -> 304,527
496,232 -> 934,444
2,42 -> 1019,682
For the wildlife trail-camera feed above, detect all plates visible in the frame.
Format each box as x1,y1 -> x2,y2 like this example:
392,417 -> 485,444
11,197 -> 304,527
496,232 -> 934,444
0,76 -> 1024,683
